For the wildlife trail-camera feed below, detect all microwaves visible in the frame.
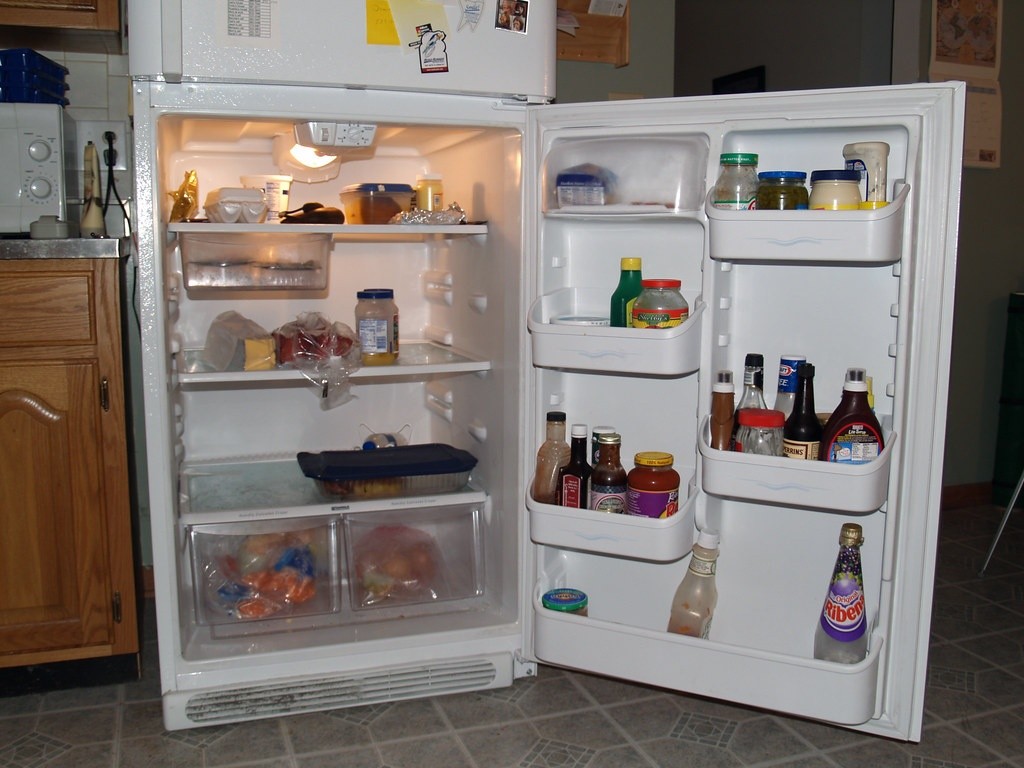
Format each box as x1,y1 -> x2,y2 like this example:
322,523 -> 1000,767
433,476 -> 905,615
0,103 -> 68,234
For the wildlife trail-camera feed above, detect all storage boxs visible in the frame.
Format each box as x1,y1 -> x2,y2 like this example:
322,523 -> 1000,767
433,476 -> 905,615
555,174 -> 611,208
340,183 -> 416,224
297,443 -> 478,499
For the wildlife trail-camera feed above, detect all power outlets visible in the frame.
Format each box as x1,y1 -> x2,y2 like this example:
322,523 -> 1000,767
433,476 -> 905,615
76,120 -> 127,170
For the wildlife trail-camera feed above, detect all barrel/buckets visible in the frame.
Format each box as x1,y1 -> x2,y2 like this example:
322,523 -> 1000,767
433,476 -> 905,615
240,173 -> 292,225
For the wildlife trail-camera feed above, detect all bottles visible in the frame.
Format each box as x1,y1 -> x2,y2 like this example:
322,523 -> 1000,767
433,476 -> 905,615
711,370 -> 735,449
533,412 -> 626,519
823,369 -> 884,464
414,173 -> 443,212
610,257 -> 642,327
714,153 -> 760,213
631,278 -> 689,330
782,362 -> 826,463
354,286 -> 402,365
363,432 -> 408,451
736,409 -> 785,456
812,523 -> 867,666
772,356 -> 806,420
665,529 -> 721,641
732,353 -> 767,455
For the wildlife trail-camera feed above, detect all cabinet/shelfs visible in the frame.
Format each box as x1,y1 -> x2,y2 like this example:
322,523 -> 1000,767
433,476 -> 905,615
0,257 -> 139,668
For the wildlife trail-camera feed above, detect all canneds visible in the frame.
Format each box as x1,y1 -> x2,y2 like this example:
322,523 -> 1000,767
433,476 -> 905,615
354,288 -> 399,366
542,588 -> 588,617
713,152 -> 861,211
415,174 -> 444,213
626,452 -> 680,519
631,279 -> 689,329
736,407 -> 786,456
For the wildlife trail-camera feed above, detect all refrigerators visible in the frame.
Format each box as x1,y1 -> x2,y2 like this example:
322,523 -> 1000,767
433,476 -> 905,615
126,0 -> 967,748
